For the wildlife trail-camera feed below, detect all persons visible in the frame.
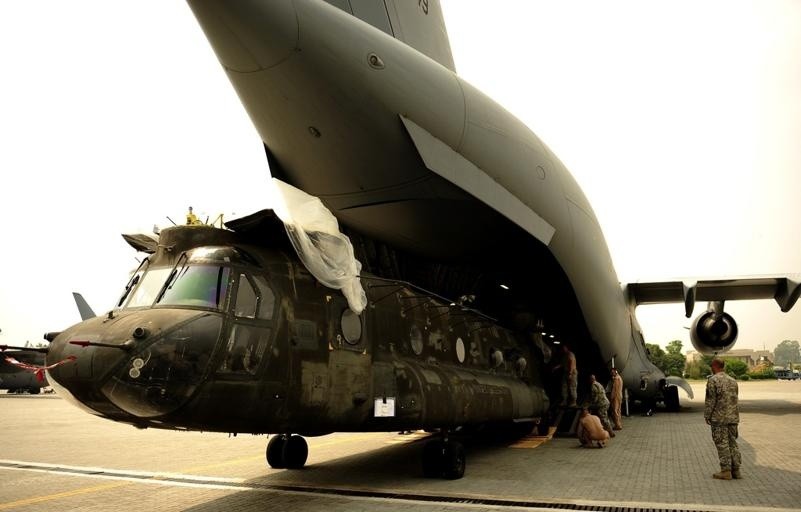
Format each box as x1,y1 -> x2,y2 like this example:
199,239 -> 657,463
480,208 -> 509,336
558,344 -> 578,407
703,358 -> 744,480
577,407 -> 610,448
607,368 -> 625,430
589,375 -> 615,438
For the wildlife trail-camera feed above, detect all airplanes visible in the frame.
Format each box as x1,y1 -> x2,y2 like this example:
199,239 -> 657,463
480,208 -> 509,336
2,348 -> 50,394
0,1 -> 801,478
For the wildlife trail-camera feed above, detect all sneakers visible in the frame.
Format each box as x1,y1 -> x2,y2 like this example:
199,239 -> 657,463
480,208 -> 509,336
584,440 -> 604,448
713,470 -> 740,479
